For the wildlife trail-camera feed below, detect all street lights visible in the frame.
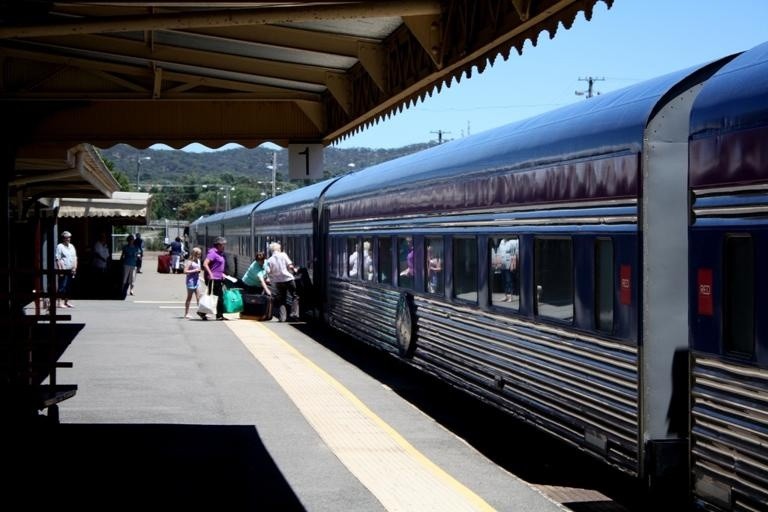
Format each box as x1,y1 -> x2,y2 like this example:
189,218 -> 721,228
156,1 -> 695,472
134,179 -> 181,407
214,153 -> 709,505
200,151 -> 285,215
347,163 -> 356,173
135,156 -> 151,191
172,206 -> 180,237
573,76 -> 607,100
429,129 -> 452,145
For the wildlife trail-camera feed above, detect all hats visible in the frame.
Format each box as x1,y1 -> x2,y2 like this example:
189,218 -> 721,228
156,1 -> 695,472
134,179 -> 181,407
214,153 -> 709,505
61,231 -> 72,238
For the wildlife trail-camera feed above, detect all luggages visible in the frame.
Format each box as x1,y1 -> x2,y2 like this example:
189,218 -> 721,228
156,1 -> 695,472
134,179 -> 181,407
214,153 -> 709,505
158,255 -> 170,273
295,268 -> 312,295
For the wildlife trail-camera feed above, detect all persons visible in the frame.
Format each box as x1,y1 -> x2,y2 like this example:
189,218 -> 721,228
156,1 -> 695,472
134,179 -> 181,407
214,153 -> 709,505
93,236 -> 111,271
427,246 -> 443,293
496,234 -> 518,304
196,236 -> 230,321
266,241 -> 300,322
119,234 -> 139,295
341,242 -> 373,281
55,230 -> 78,308
182,246 -> 203,319
134,232 -> 145,273
397,235 -> 415,279
236,249 -> 272,296
165,237 -> 182,275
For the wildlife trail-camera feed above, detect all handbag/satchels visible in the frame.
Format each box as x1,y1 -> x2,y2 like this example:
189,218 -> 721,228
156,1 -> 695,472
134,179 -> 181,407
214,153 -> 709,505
197,280 -> 271,321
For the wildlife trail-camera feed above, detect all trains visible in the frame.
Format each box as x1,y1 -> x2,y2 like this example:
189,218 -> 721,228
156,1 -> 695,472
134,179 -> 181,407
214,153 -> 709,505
186,37 -> 767,512
